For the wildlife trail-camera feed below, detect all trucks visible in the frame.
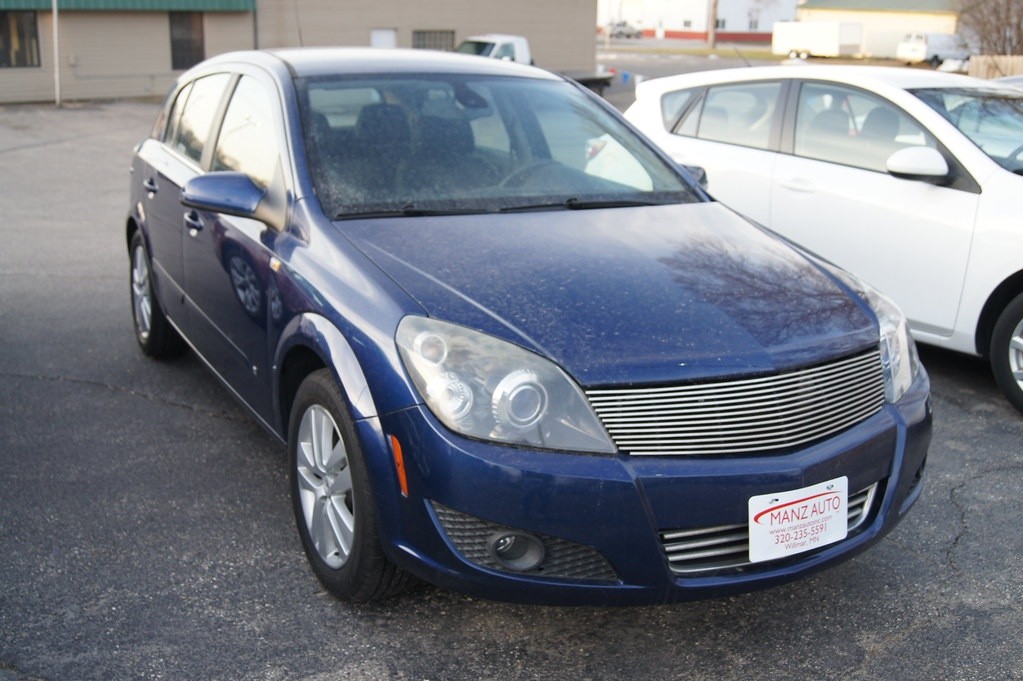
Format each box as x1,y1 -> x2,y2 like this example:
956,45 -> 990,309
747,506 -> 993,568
450,32 -> 615,103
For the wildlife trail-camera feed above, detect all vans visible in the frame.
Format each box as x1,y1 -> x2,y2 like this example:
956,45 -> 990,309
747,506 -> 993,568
898,32 -> 972,65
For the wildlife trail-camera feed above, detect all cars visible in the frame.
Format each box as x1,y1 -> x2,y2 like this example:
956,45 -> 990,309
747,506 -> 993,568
208,211 -> 315,326
850,74 -> 1022,158
607,22 -> 643,39
124,48 -> 935,608
579,63 -> 1023,418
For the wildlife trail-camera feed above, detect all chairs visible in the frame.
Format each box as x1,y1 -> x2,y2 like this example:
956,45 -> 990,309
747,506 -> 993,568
307,112 -> 375,206
862,107 -> 899,144
391,115 -> 505,201
354,102 -> 412,207
699,106 -> 727,135
805,108 -> 851,148
